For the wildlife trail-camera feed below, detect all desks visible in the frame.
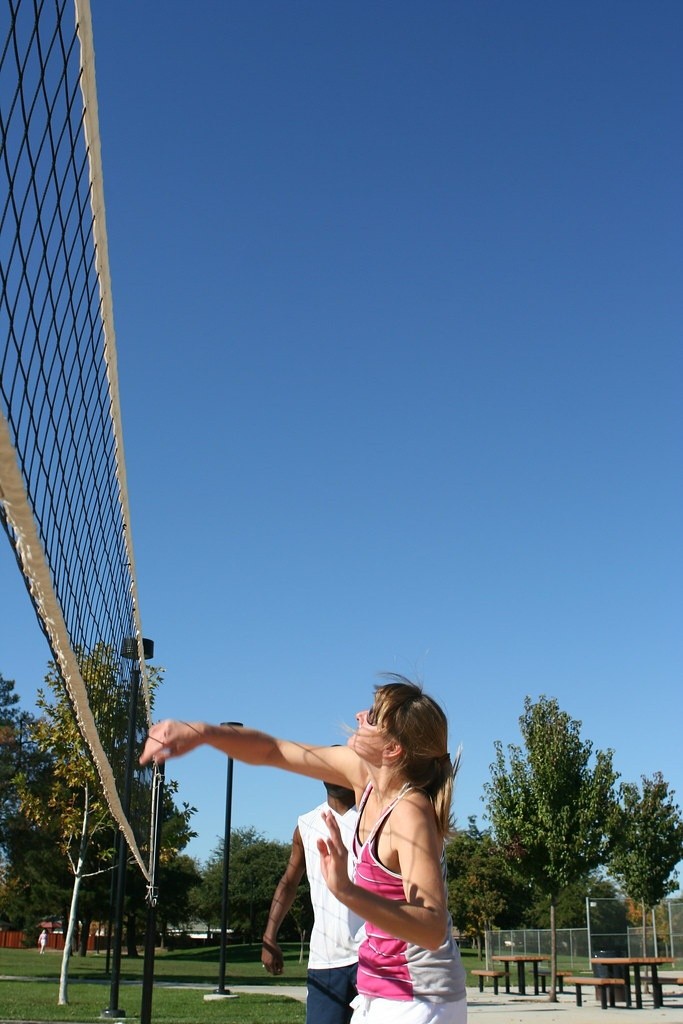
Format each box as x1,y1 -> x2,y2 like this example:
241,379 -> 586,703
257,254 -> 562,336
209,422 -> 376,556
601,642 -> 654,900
491,955 -> 550,994
590,956 -> 674,1010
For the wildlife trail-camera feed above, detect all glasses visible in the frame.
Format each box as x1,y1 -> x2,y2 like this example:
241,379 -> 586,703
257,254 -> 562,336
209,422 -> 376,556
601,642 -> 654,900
366,706 -> 385,728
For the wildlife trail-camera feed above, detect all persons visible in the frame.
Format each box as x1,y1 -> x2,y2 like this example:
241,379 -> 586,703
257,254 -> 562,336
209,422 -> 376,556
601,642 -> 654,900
261,744 -> 368,1024
38,930 -> 47,955
138,682 -> 467,1024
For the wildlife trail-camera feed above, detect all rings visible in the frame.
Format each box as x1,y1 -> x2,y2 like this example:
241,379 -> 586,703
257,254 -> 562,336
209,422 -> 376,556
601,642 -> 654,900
262,965 -> 265,968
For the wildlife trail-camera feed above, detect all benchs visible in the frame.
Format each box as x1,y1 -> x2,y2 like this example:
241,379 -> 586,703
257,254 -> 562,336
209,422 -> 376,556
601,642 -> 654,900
529,970 -> 573,993
563,976 -> 626,1009
640,976 -> 683,997
470,969 -> 511,995
